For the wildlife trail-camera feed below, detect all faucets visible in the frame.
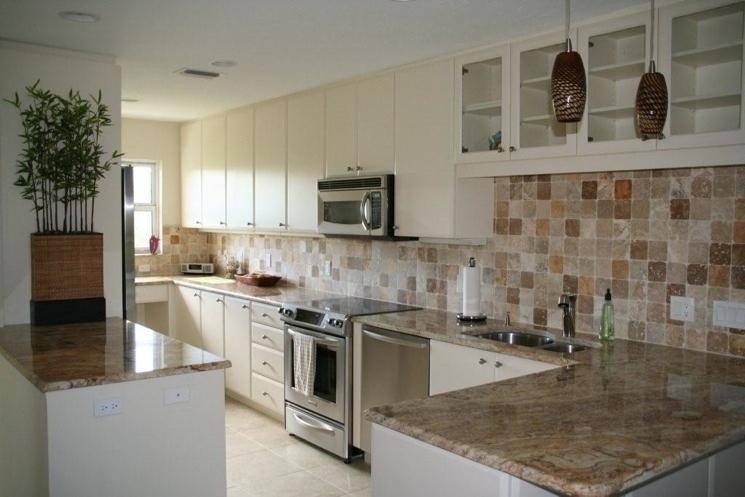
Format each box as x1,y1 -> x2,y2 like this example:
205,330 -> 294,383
557,294 -> 577,339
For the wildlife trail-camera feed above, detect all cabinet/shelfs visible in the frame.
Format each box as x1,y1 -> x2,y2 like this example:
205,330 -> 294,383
453,0 -> 745,165
429,340 -> 563,398
168,282 -> 284,428
0,316 -> 231,497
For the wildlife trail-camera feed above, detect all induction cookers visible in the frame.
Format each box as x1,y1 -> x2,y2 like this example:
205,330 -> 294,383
279,294 -> 424,336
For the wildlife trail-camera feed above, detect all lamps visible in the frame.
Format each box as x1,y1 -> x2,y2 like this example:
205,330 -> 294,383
634,0 -> 668,141
550,0 -> 586,122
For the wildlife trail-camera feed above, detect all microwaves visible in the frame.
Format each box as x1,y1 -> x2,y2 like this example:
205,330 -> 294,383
315,174 -> 419,241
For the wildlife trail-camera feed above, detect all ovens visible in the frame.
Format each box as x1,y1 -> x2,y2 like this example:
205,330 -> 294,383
281,322 -> 364,462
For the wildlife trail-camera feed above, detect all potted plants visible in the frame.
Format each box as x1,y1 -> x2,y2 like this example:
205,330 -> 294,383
2,78 -> 125,325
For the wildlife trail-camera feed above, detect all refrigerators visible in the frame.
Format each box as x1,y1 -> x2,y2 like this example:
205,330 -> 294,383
121,164 -> 137,322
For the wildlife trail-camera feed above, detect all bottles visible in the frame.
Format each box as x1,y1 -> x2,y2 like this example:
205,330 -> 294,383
599,288 -> 615,341
600,340 -> 614,390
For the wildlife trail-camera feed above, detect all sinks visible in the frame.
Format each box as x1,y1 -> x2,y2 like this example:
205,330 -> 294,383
474,331 -> 555,348
543,342 -> 586,354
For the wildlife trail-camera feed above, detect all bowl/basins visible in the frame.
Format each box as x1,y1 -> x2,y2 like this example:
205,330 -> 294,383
232,273 -> 282,286
